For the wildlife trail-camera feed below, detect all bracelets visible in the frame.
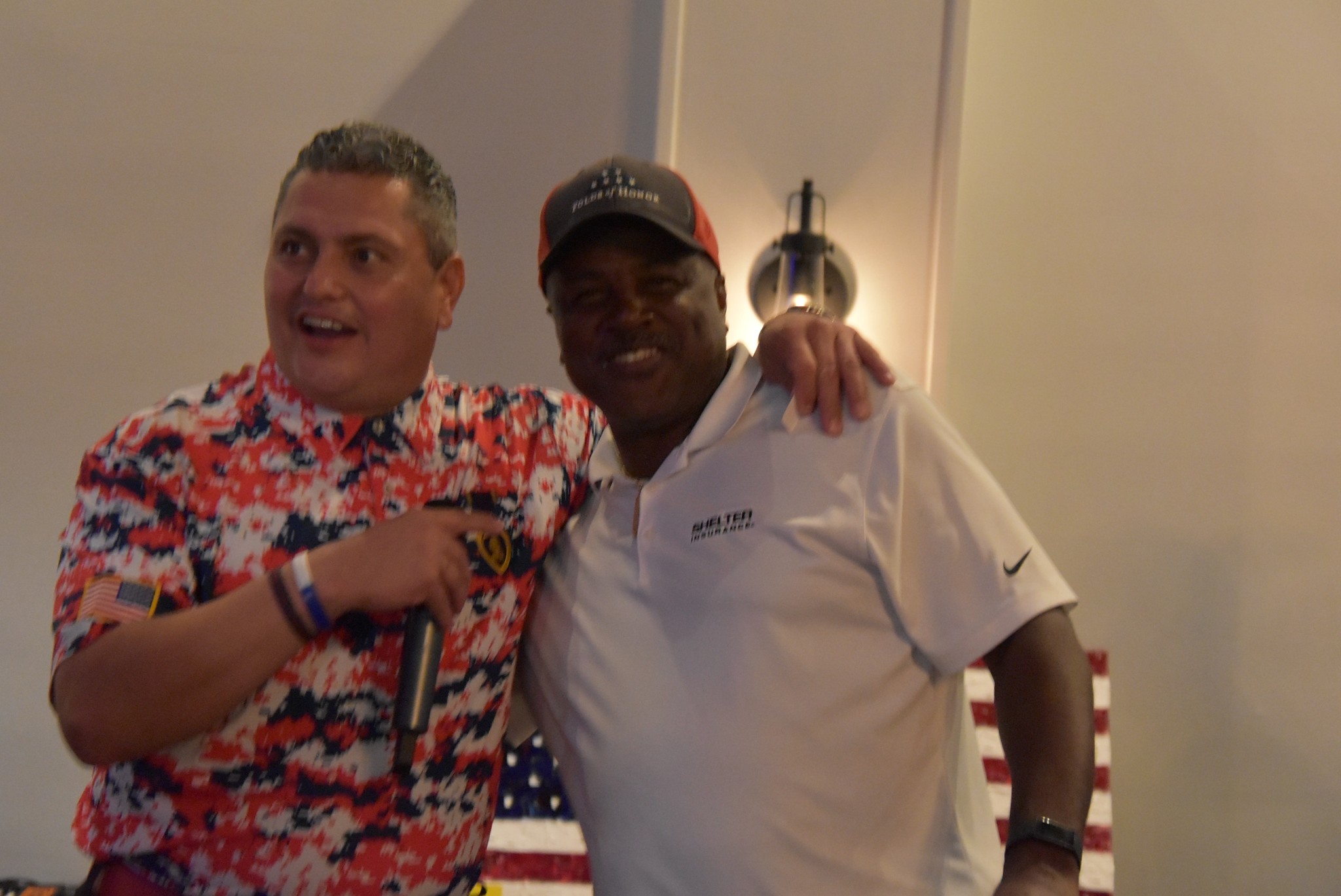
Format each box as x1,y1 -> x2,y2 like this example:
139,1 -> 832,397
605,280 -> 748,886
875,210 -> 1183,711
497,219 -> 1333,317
293,550 -> 330,632
1005,814 -> 1083,867
270,569 -> 308,644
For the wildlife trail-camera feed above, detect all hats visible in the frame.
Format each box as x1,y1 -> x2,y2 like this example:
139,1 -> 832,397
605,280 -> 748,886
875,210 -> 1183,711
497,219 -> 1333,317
535,157 -> 721,287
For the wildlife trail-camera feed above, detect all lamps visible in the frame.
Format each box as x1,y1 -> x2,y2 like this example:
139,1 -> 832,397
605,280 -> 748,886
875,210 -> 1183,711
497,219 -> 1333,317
749,181 -> 859,324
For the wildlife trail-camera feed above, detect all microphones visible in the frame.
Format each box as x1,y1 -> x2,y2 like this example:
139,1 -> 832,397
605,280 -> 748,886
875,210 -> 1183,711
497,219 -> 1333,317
390,500 -> 464,778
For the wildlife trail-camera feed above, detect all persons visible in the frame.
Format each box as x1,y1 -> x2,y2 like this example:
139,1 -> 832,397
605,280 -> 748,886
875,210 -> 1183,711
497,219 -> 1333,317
496,154 -> 1093,896
49,121 -> 894,895
